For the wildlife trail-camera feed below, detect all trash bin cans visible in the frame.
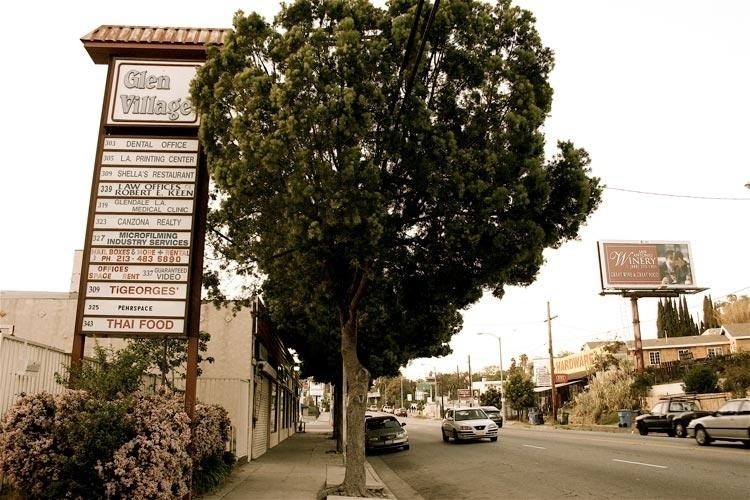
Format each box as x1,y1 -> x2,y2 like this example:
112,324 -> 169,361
559,413 -> 568,425
529,407 -> 544,425
618,409 -> 631,427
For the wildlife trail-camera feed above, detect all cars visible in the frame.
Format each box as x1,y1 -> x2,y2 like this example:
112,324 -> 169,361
364,415 -> 410,452
686,398 -> 750,447
439,406 -> 502,442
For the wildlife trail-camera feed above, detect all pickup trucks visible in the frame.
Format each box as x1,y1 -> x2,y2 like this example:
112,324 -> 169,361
635,396 -> 713,437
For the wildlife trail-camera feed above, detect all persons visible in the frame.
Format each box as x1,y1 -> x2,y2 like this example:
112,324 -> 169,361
673,251 -> 691,281
662,251 -> 675,283
